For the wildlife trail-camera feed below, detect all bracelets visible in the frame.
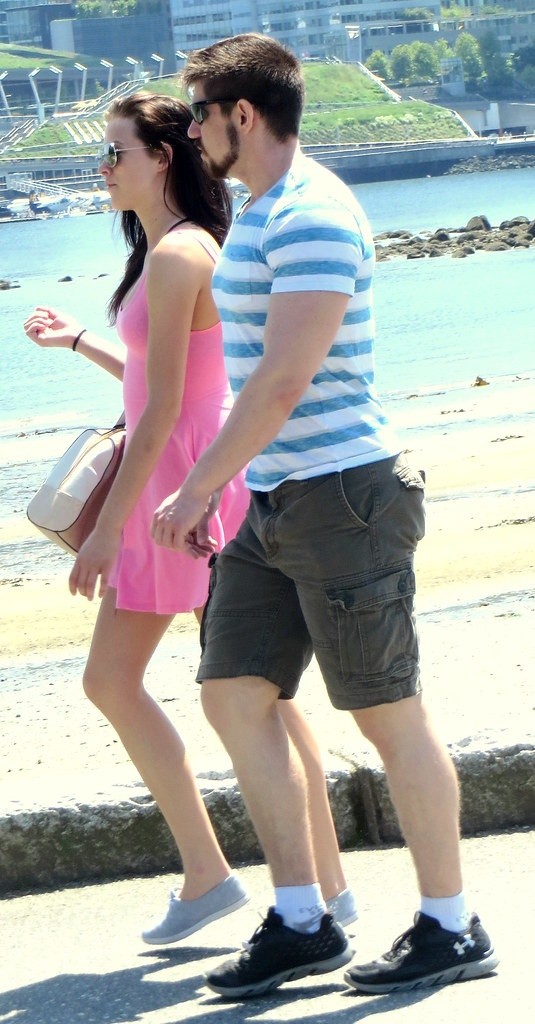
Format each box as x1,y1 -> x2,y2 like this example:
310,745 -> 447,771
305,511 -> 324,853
72,328 -> 87,352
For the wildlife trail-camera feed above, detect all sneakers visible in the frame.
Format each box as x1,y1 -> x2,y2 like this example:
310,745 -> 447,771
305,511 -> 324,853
324,886 -> 359,930
343,909 -> 500,994
141,875 -> 250,945
202,904 -> 357,998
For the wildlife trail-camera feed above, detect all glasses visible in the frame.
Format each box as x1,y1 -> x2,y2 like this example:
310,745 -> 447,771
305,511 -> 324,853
188,99 -> 235,124
99,141 -> 155,169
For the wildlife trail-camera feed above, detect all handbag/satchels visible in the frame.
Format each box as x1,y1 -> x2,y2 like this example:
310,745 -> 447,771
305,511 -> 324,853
26,422 -> 127,558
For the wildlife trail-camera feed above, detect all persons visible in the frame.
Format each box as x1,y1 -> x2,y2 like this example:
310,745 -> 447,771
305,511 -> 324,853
22,90 -> 365,948
146,33 -> 500,996
